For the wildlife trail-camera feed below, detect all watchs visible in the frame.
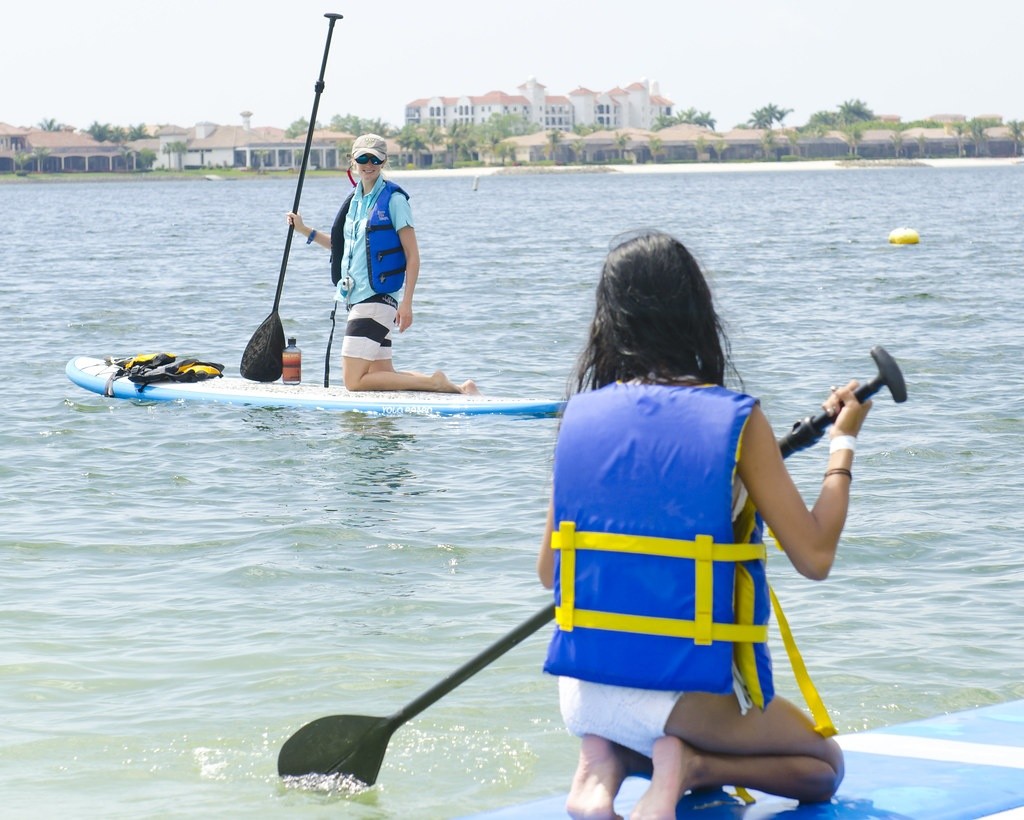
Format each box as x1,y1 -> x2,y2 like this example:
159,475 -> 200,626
307,228 -> 317,245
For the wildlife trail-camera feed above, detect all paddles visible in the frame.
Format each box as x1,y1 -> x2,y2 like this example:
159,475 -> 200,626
272,344 -> 912,787
237,10 -> 346,383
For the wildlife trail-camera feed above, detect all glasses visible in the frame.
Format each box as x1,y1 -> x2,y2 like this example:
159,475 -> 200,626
354,154 -> 383,165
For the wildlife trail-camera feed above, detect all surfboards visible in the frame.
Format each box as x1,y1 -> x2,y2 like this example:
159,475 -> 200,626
483,695 -> 1024,820
64,351 -> 568,420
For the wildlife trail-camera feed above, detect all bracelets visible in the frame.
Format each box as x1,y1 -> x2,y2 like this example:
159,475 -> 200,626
825,467 -> 852,479
830,435 -> 855,453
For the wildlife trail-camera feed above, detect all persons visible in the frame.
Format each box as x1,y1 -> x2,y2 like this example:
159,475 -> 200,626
287,132 -> 481,394
541,235 -> 874,820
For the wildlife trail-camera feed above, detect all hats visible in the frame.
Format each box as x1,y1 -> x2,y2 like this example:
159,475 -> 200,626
352,133 -> 388,160
